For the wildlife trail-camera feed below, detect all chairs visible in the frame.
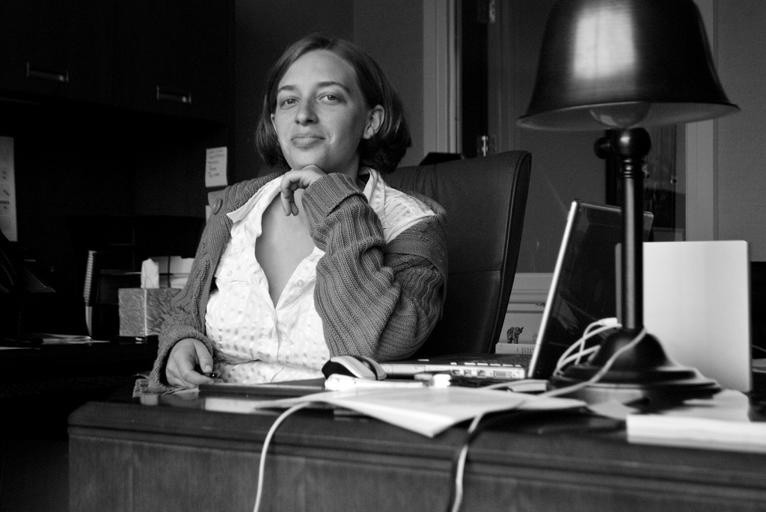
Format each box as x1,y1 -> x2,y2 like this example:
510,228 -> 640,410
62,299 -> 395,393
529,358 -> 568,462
375,150 -> 533,357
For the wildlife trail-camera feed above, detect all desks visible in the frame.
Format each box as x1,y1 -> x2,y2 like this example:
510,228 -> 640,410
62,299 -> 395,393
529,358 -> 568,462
66,384 -> 766,510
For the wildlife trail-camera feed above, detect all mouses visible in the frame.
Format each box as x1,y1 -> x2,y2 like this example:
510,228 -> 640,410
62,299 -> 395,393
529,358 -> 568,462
321,347 -> 388,383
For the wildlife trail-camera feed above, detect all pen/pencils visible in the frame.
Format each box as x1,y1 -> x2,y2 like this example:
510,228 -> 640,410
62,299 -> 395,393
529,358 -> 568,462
202,373 -> 217,377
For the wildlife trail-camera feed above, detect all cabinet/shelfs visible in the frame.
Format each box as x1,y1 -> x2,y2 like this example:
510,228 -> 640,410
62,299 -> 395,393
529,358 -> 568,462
0,1 -> 238,511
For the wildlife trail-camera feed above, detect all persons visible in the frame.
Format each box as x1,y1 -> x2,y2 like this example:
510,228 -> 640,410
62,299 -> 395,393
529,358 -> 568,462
144,33 -> 451,403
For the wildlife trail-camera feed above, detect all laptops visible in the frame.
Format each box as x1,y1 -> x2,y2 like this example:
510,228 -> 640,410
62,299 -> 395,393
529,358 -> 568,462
378,198 -> 655,381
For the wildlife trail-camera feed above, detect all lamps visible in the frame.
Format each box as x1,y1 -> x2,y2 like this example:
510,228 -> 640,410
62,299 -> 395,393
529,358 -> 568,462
517,0 -> 743,410
